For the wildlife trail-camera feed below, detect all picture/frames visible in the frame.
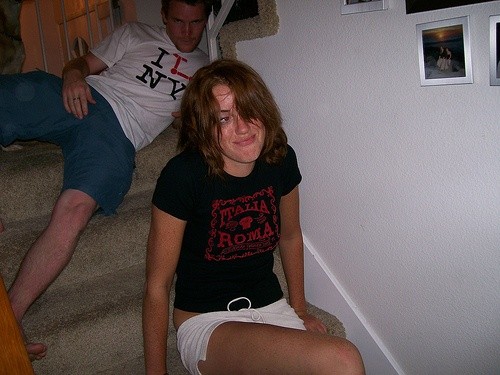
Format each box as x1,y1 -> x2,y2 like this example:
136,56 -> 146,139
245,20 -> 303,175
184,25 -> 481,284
340,0 -> 388,15
489,15 -> 500,85
405,0 -> 496,14
416,15 -> 473,87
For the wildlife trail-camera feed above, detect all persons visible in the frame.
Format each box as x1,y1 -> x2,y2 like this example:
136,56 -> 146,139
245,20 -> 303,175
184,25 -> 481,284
436,45 -> 451,71
0,0 -> 214,363
140,58 -> 369,375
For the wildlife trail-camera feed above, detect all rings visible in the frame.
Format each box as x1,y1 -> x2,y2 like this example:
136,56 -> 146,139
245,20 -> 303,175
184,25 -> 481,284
73,98 -> 80,100
